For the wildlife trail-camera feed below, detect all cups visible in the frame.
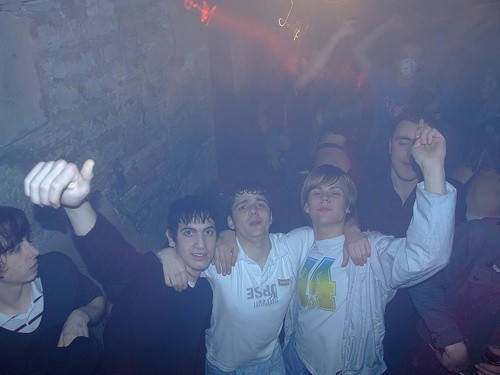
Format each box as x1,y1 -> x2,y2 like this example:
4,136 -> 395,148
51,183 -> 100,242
481,344 -> 500,365
